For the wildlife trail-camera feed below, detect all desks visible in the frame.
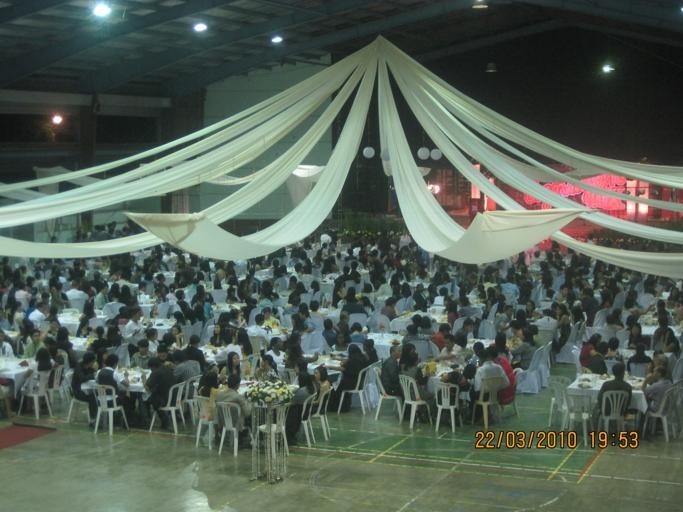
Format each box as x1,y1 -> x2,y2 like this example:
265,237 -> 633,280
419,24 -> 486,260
248,401 -> 290,486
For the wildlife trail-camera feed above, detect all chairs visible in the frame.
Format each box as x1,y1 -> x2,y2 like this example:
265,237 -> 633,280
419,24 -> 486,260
539,341 -> 553,388
471,376 -> 505,432
18,369 -> 54,419
312,387 -> 335,440
435,382 -> 464,433
181,375 -> 200,426
92,384 -> 130,436
337,360 -> 382,415
149,381 -> 185,434
300,393 -> 316,448
375,367 -> 403,423
547,376 -> 574,427
642,385 -> 679,442
499,367 -> 523,417
564,388 -> 600,446
399,375 -> 433,429
598,390 -> 630,433
64,369 -> 92,424
216,402 -> 245,456
516,345 -> 544,394
48,366 -> 64,410
192,396 -> 222,450
